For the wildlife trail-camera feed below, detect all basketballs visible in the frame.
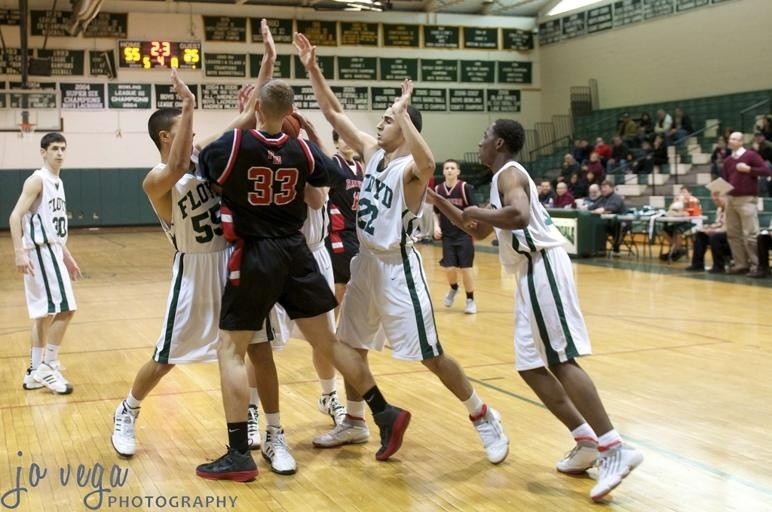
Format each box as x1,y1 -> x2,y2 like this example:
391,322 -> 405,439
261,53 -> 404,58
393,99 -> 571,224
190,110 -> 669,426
282,114 -> 300,139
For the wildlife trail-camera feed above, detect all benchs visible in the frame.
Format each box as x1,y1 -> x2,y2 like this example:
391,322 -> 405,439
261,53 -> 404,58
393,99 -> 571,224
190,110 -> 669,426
469,89 -> 772,276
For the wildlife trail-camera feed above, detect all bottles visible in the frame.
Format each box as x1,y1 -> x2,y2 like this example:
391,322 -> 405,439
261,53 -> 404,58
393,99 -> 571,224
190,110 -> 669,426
549,197 -> 553,208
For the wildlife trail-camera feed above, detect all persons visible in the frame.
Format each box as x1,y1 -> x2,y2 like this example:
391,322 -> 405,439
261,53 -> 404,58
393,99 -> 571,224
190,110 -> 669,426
9,133 -> 82,393
108,18 -> 771,501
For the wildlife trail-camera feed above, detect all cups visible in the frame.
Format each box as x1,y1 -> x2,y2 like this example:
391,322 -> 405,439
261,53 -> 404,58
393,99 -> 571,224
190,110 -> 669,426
756,198 -> 763,212
688,200 -> 697,214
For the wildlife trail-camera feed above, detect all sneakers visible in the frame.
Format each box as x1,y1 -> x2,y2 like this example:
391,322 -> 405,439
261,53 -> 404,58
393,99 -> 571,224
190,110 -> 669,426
660,247 -> 766,279
590,438 -> 645,499
194,446 -> 259,482
22,360 -> 74,396
557,436 -> 601,474
110,398 -> 142,458
372,402 -> 412,461
316,389 -> 348,428
260,423 -> 298,476
444,283 -> 459,307
245,403 -> 262,449
468,403 -> 511,465
463,298 -> 477,314
311,414 -> 372,449
